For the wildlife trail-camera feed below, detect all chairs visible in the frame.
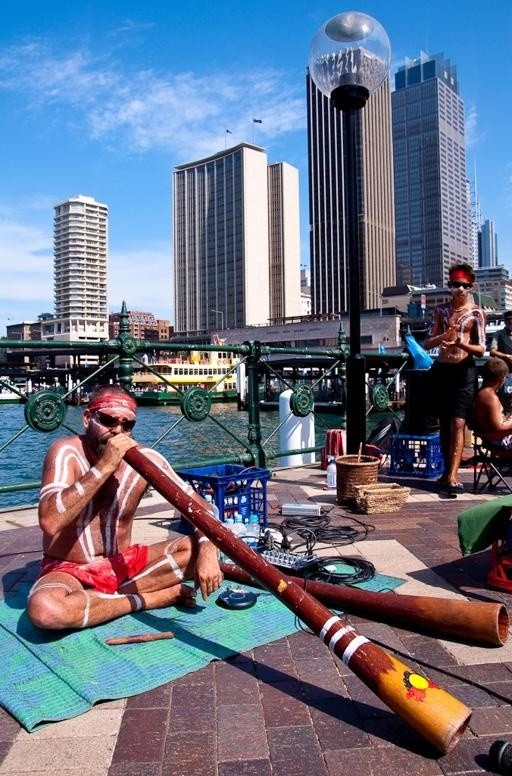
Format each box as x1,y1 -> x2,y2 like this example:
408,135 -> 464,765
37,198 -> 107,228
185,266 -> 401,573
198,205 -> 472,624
472,429 -> 512,492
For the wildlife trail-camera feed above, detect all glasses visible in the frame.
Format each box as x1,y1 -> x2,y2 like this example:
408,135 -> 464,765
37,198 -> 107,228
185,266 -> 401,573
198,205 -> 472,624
451,281 -> 473,290
95,409 -> 137,432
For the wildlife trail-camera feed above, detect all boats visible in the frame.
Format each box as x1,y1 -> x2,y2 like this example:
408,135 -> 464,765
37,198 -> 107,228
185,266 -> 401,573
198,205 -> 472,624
0,372 -> 89,405
132,334 -> 237,406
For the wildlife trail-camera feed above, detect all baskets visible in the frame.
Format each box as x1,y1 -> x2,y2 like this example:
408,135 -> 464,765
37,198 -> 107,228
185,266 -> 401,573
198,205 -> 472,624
174,463 -> 270,534
354,482 -> 411,515
335,441 -> 381,506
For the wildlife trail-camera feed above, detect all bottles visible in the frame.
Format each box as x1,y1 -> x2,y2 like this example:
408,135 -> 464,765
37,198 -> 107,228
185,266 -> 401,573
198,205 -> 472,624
203,494 -> 261,566
326,460 -> 338,489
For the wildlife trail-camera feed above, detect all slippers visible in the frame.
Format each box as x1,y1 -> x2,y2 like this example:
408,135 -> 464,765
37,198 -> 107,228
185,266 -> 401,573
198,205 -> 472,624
438,478 -> 464,491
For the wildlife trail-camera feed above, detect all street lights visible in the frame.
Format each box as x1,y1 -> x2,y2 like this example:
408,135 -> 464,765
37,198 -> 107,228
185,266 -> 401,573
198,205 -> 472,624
305,9 -> 395,455
211,308 -> 224,331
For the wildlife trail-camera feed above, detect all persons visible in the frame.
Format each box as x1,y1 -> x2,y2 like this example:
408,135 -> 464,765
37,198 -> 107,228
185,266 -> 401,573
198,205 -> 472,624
424,263 -> 486,492
468,358 -> 512,451
490,311 -> 511,416
27,385 -> 224,630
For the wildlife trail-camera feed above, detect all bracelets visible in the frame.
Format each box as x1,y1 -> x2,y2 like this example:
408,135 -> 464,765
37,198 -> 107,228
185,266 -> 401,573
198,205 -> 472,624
504,354 -> 509,360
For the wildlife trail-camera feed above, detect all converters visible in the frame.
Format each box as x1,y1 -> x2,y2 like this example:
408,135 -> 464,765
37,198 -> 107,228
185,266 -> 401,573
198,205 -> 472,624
281,503 -> 322,517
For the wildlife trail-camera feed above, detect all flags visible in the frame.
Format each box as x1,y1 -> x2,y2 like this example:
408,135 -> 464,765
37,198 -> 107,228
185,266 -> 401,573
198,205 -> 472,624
253,119 -> 262,123
226,129 -> 232,134
